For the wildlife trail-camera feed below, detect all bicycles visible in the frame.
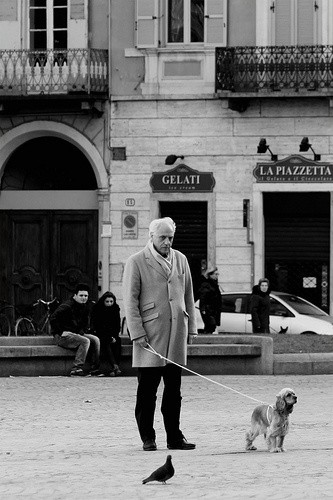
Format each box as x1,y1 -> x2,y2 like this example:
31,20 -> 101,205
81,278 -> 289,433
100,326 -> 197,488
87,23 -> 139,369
0,296 -> 63,337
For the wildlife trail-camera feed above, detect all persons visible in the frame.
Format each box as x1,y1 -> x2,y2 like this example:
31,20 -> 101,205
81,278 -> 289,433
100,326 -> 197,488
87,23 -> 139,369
123,217 -> 198,451
90,292 -> 122,376
49,283 -> 104,377
199,267 -> 224,335
249,278 -> 273,335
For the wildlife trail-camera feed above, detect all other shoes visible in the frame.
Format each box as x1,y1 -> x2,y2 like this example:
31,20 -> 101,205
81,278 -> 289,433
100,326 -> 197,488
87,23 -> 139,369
91,369 -> 104,376
71,369 -> 90,377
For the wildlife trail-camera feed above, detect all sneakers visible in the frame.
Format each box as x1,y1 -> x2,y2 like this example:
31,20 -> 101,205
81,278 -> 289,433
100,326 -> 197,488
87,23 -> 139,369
167,437 -> 197,450
143,439 -> 157,450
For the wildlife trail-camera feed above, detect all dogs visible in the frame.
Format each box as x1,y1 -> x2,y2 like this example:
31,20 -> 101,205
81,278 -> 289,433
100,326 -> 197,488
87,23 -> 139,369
244,386 -> 298,454
276,325 -> 288,334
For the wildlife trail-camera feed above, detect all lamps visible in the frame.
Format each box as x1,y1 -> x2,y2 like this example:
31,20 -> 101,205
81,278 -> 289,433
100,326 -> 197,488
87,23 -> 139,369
165,155 -> 184,166
299,137 -> 321,161
257,138 -> 278,161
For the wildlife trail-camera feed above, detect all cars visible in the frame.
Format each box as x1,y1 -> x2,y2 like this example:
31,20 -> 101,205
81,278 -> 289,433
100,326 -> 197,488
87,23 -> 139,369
193,291 -> 333,336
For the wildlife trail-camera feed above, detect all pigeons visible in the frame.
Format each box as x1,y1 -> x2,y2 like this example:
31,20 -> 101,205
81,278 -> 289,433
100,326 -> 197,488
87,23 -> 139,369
141,454 -> 176,486
